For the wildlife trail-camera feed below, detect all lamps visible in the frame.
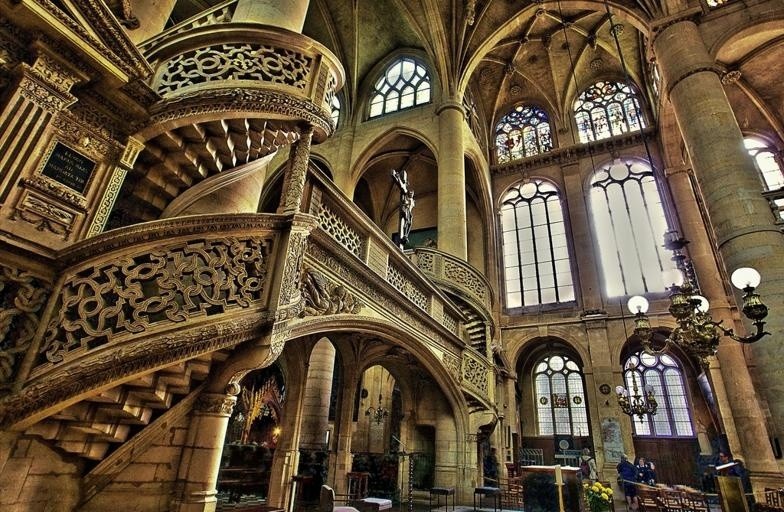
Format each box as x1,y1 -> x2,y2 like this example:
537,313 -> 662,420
555,2 -> 773,424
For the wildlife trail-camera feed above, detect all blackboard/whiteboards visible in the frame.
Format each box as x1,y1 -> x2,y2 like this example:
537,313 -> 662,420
40,141 -> 96,195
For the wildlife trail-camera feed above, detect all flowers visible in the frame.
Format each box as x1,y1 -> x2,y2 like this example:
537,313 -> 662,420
583,480 -> 613,511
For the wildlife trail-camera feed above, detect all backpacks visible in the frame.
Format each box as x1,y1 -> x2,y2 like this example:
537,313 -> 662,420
580,457 -> 592,476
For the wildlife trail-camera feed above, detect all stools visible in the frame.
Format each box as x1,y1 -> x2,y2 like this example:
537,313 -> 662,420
429,487 -> 455,511
360,496 -> 392,511
473,486 -> 501,511
650,482 -> 709,512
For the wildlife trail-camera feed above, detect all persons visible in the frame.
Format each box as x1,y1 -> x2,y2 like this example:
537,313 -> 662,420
616,453 -> 656,511
579,448 -> 596,484
717,451 -> 750,491
395,173 -> 415,243
487,448 -> 501,480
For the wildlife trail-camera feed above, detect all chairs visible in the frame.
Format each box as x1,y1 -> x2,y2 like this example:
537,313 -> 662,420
497,476 -> 523,510
318,485 -> 364,512
